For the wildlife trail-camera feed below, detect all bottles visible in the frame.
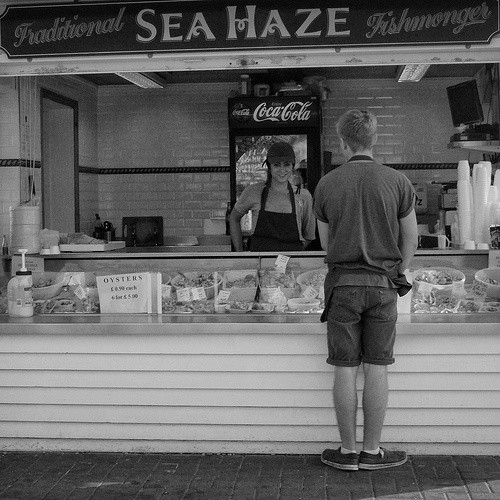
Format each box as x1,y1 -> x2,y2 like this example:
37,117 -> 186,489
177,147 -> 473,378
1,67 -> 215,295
2,235 -> 9,255
93,213 -> 115,241
238,75 -> 271,97
225,202 -> 231,235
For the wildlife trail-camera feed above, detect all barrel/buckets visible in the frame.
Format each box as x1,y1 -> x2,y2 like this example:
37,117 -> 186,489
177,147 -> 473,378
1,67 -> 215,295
41,229 -> 60,249
10,206 -> 43,254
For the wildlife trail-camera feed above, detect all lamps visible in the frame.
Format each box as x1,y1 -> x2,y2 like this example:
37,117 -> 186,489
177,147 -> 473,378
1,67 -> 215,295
394,64 -> 431,83
114,72 -> 166,89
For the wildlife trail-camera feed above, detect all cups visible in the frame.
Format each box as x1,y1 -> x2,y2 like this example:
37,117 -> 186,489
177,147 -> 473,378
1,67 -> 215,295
438,235 -> 451,249
457,161 -> 500,250
323,150 -> 333,173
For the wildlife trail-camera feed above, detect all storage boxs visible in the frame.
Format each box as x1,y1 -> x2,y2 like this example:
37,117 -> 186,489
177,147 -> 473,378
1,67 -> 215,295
413,183 -> 428,214
438,186 -> 458,226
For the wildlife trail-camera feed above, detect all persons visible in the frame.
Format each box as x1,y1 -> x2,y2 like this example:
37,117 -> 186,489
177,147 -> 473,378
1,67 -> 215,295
310,109 -> 422,471
228,141 -> 317,251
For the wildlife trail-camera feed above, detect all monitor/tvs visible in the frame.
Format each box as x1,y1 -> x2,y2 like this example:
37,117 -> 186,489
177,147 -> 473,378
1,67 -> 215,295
446,79 -> 484,129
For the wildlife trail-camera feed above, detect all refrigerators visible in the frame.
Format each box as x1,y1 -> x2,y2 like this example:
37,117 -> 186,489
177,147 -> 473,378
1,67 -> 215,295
228,95 -> 326,250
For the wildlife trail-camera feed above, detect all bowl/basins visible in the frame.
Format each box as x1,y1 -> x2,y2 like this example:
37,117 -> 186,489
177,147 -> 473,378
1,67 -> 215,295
32,270 -> 328,314
40,246 -> 61,254
412,266 -> 500,314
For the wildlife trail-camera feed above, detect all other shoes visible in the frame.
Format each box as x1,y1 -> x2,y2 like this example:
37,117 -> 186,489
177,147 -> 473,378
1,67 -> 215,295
320,445 -> 359,471
358,447 -> 408,470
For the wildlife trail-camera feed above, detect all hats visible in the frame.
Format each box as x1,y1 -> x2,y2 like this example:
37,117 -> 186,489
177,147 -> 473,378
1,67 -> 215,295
267,141 -> 296,165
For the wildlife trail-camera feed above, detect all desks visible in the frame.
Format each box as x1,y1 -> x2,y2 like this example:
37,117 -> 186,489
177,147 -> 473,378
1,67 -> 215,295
416,214 -> 440,249
113,236 -> 232,252
